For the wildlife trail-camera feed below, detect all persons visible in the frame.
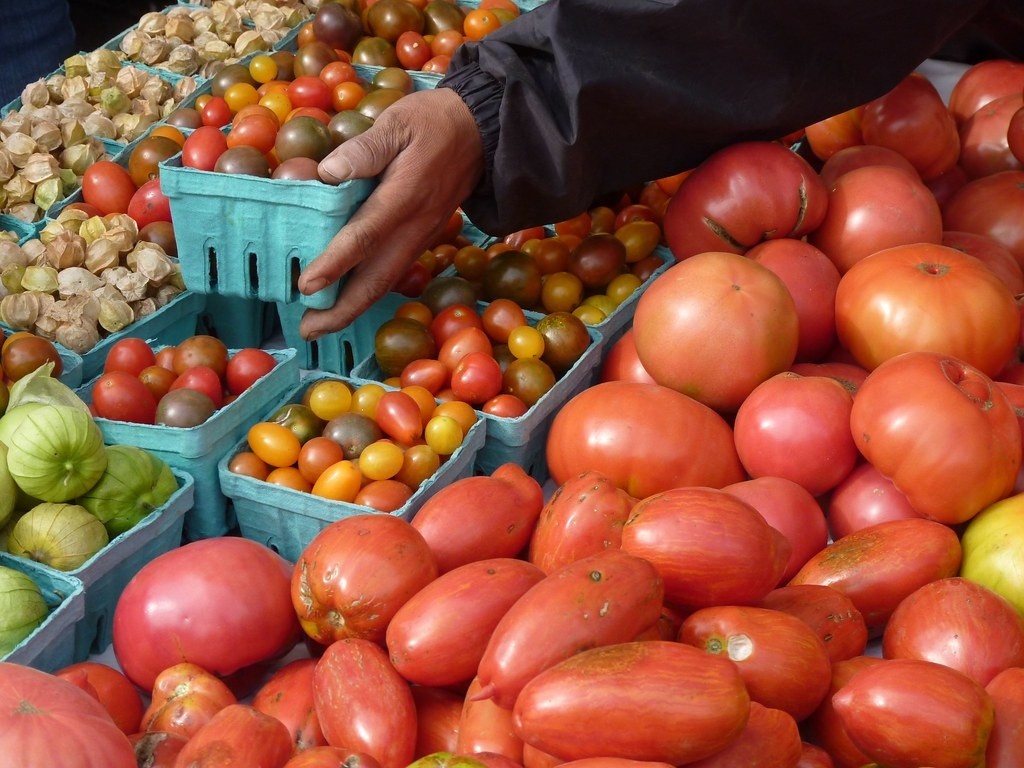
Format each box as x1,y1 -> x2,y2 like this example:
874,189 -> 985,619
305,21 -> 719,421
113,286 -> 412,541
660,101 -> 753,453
297,0 -> 1024,343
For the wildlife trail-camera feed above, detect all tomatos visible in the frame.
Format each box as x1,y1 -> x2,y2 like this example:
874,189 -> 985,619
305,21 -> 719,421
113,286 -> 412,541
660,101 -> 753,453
1,0 -> 1024,766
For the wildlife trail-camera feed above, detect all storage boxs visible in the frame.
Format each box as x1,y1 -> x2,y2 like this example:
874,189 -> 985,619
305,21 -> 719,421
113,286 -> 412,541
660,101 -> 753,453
19,467 -> 194,664
44,122 -> 280,349
475,226 -> 675,356
0,552 -> 86,673
350,302 -> 604,488
218,371 -> 487,564
71,341 -> 302,541
97,4 -> 256,84
0,51 -> 205,166
0,291 -> 198,384
273,0 -> 527,81
158,67 -> 441,311
